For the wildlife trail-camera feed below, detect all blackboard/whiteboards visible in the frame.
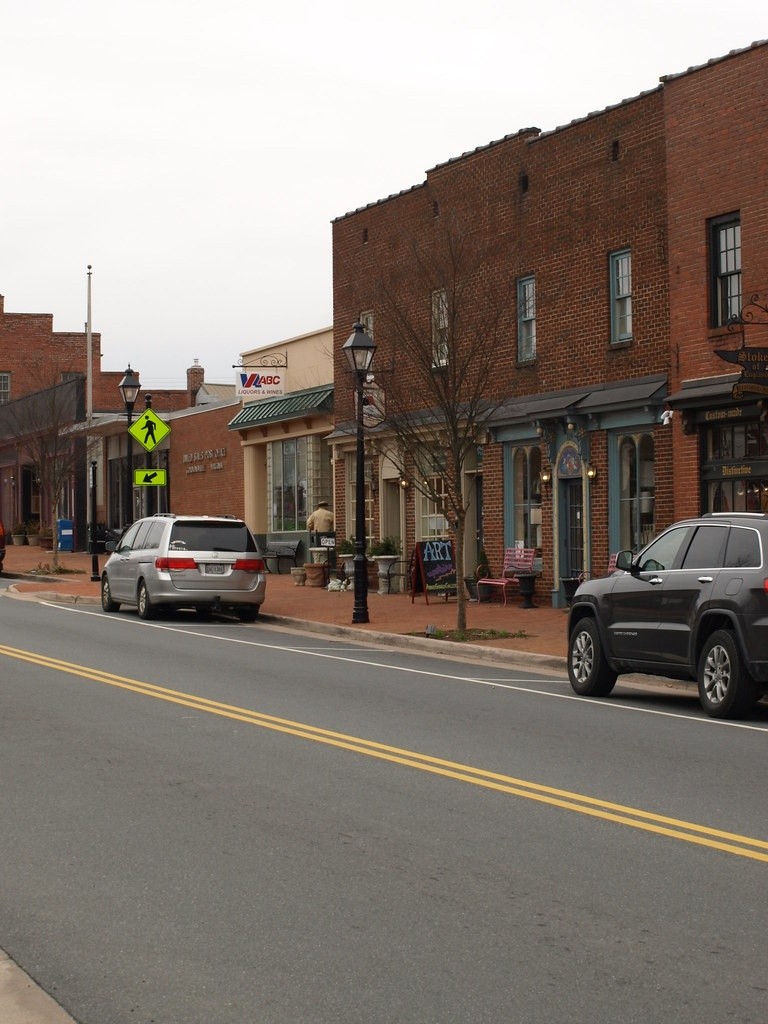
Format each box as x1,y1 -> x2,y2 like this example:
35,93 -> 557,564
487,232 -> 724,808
413,539 -> 458,593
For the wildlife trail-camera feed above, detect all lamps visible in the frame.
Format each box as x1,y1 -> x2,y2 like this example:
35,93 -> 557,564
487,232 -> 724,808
539,467 -> 550,485
370,477 -> 377,494
10,476 -> 16,488
398,472 -> 409,490
586,461 -> 597,482
36,475 -> 41,486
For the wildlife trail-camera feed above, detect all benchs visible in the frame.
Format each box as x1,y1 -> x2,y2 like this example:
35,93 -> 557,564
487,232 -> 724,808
474,546 -> 538,605
261,539 -> 303,575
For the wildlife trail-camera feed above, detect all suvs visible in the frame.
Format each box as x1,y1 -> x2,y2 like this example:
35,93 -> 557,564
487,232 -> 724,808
99,513 -> 268,622
565,511 -> 768,720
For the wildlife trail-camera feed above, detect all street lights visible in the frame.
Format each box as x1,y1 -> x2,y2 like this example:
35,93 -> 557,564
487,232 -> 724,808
115,361 -> 143,535
341,322 -> 381,623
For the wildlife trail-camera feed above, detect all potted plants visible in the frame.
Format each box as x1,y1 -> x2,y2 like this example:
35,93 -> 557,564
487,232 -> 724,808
337,536 -> 357,590
370,537 -> 400,593
11,521 -> 26,546
40,527 -> 53,549
26,520 -> 40,546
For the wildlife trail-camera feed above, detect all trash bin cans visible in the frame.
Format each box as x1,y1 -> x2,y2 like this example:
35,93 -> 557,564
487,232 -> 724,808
56,518 -> 74,551
87,521 -> 106,554
308,529 -> 336,568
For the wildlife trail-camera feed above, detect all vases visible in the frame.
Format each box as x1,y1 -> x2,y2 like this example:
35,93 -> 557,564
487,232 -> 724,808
307,546 -> 334,564
303,563 -> 331,587
290,567 -> 306,586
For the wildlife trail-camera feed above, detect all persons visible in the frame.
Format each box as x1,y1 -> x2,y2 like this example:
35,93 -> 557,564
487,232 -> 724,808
306,501 -> 335,533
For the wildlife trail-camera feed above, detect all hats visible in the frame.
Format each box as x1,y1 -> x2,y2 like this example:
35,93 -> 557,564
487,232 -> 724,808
318,501 -> 329,507
